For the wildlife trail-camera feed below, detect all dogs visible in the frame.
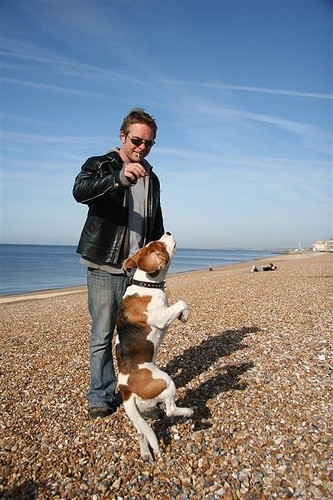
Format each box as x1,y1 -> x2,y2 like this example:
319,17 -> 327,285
115,232 -> 194,462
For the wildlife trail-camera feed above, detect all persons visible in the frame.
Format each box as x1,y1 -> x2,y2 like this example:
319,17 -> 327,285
209,262 -> 212,271
251,263 -> 277,272
73,110 -> 165,417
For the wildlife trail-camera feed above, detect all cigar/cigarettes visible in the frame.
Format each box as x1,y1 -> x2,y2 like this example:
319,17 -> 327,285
137,152 -> 140,159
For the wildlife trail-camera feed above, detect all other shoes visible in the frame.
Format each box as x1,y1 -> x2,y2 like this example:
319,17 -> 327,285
88,403 -> 112,418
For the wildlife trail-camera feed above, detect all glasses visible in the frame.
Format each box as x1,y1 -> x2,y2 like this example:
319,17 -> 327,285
125,134 -> 155,147
